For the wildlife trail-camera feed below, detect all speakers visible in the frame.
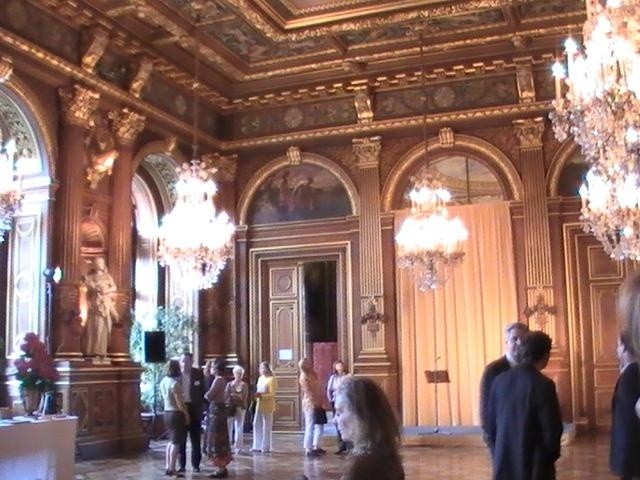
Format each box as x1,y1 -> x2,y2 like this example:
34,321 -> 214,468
144,331 -> 166,363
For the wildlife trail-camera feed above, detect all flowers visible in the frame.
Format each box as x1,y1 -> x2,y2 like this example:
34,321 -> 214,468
13,333 -> 61,387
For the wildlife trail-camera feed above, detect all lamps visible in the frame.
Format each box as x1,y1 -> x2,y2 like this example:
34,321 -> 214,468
545,1 -> 640,267
144,331 -> 167,441
154,1 -> 240,292
43,267 -> 63,360
392,0 -> 473,290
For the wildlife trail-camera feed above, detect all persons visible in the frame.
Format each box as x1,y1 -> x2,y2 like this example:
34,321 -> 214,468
204,358 -> 234,479
479,322 -> 529,460
614,269 -> 640,361
326,360 -> 350,455
487,330 -> 564,480
226,365 -> 249,455
202,361 -> 214,432
82,257 -> 118,359
608,329 -> 640,480
159,359 -> 191,476
298,357 -> 329,457
248,361 -> 278,453
177,351 -> 210,472
333,373 -> 405,480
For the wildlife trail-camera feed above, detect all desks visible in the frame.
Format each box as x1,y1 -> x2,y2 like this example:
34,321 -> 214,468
0,414 -> 78,480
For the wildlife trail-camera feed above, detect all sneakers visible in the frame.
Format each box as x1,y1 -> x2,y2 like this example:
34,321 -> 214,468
231,447 -> 273,456
164,466 -> 230,479
304,450 -> 320,458
316,447 -> 326,454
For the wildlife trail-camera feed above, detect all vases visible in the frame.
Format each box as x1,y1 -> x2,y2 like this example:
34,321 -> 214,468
19,384 -> 41,418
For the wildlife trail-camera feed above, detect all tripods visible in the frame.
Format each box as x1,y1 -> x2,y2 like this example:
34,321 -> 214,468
146,365 -> 166,440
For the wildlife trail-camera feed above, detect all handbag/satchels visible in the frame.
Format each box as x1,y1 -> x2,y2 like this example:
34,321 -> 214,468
313,407 -> 327,424
223,406 -> 237,418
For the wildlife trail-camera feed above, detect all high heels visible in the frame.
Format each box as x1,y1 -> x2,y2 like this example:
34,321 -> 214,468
335,449 -> 346,454
